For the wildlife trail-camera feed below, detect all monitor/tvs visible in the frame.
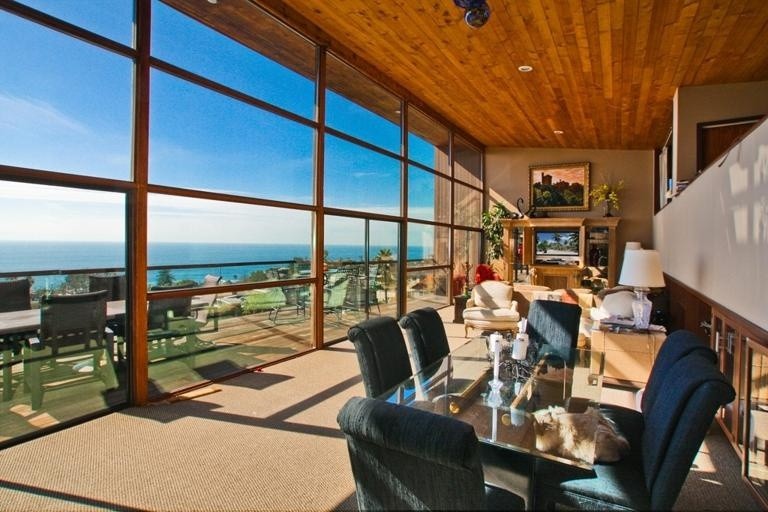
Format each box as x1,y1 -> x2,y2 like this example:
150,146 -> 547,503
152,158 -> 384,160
534,231 -> 580,263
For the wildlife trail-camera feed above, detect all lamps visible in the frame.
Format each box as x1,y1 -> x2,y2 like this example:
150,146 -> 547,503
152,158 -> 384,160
617,248 -> 667,333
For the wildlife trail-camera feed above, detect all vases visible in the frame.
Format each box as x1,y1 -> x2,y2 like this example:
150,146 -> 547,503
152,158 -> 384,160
603,200 -> 613,217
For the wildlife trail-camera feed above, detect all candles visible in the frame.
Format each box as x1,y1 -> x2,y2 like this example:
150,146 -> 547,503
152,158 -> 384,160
488,391 -> 501,444
490,335 -> 501,381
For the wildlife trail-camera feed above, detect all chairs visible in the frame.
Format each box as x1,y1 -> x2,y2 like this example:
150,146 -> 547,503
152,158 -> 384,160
88,274 -> 128,359
533,329 -> 736,512
197,275 -> 222,332
400,306 -> 451,383
522,301 -> 583,369
107,282 -> 194,369
336,394 -> 524,512
268,270 -> 305,322
347,261 -> 380,318
347,316 -> 413,400
24,290 -> 119,410
0,279 -> 37,402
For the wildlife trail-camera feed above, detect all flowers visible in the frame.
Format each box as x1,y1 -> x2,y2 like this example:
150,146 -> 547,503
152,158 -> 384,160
589,176 -> 626,213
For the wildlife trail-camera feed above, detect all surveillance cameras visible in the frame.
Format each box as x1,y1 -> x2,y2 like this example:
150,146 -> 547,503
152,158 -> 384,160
442,0 -> 488,32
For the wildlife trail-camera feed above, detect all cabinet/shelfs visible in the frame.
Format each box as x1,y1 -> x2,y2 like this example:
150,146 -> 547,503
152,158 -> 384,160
588,319 -> 668,393
498,214 -> 621,291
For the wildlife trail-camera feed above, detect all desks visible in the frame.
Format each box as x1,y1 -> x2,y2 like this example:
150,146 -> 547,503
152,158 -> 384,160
0,296 -> 208,336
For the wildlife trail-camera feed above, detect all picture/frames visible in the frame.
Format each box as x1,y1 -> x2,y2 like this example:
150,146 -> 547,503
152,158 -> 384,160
526,159 -> 591,211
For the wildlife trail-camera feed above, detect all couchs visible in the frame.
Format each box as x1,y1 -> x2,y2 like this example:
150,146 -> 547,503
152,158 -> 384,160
461,279 -> 522,338
